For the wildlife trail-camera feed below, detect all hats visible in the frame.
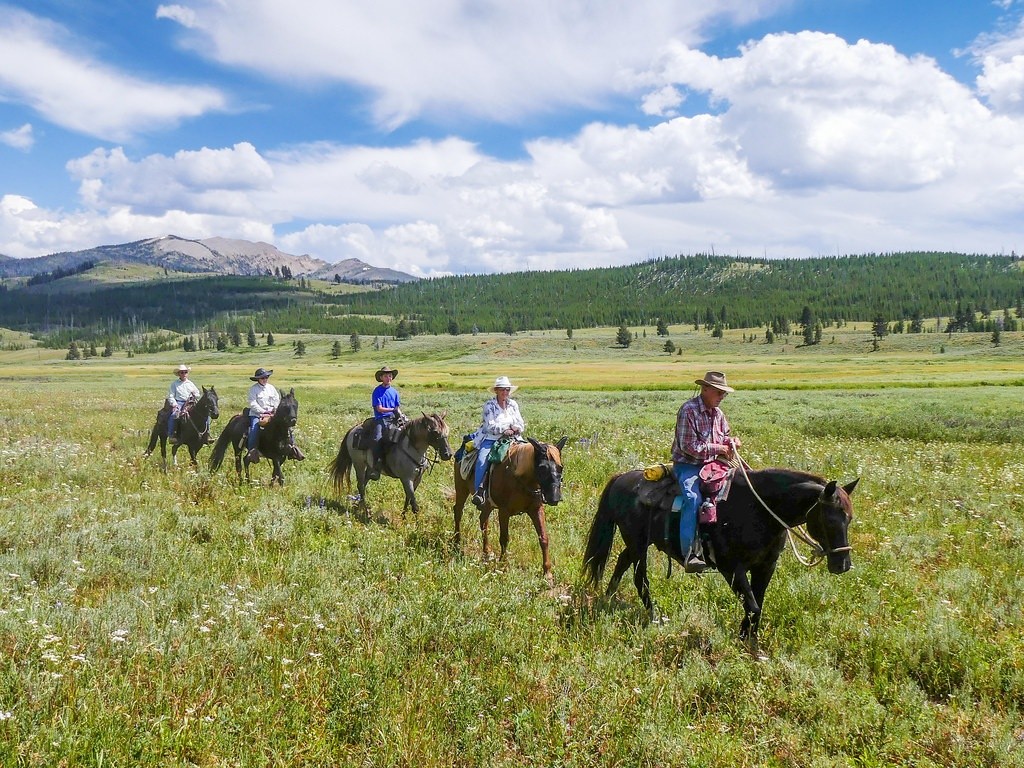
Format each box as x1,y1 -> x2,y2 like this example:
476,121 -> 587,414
173,364 -> 191,376
375,367 -> 398,382
695,371 -> 735,393
487,376 -> 518,395
250,368 -> 273,381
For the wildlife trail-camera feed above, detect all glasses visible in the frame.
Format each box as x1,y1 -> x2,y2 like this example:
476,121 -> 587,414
709,386 -> 729,397
181,372 -> 188,374
498,387 -> 510,391
261,376 -> 268,380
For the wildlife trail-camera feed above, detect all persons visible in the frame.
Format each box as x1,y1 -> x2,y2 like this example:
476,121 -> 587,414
370,366 -> 409,480
471,376 -> 524,505
167,364 -> 200,444
247,368 -> 295,459
670,371 -> 742,574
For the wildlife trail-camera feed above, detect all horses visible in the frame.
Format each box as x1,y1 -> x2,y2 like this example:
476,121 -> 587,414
209,388 -> 298,485
142,384 -> 220,466
453,436 -> 568,590
323,411 -> 453,525
581,469 -> 861,640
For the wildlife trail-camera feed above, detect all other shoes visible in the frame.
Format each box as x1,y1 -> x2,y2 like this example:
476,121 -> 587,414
473,495 -> 484,510
208,439 -> 214,443
169,437 -> 178,444
685,556 -> 706,573
370,471 -> 380,480
250,448 -> 259,464
